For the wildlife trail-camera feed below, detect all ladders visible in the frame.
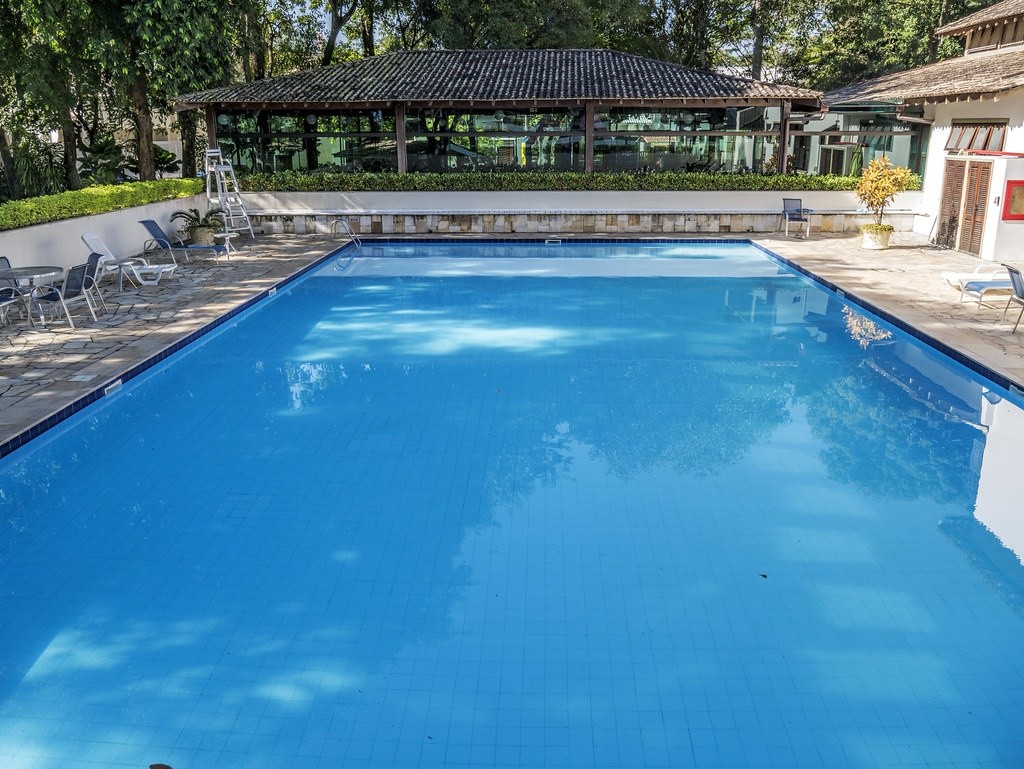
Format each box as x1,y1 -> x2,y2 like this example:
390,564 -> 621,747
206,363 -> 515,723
205,146 -> 255,239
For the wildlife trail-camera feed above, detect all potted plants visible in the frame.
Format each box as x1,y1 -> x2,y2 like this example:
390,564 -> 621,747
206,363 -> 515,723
169,207 -> 226,245
853,155 -> 912,250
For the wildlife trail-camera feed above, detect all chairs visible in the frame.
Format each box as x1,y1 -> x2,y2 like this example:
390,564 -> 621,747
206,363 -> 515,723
0,286 -> 36,328
778,198 -> 811,237
959,281 -> 1014,311
0,256 -> 46,326
49,251 -> 110,315
30,263 -> 97,329
81,232 -> 177,286
941,264 -> 1010,292
1000,263 -> 1024,334
138,218 -> 230,266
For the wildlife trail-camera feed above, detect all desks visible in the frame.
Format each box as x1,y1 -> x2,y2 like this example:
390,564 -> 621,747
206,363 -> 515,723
0,266 -> 64,328
104,258 -> 137,293
